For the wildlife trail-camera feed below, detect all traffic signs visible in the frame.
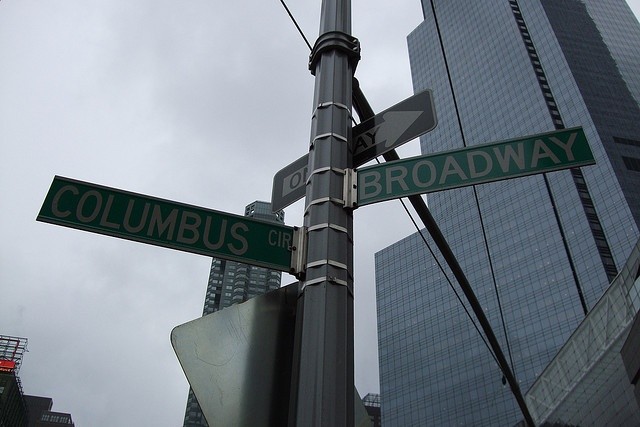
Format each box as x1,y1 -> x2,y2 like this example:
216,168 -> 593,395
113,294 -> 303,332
270,88 -> 438,215
170,280 -> 375,426
357,126 -> 597,208
35,175 -> 294,273
